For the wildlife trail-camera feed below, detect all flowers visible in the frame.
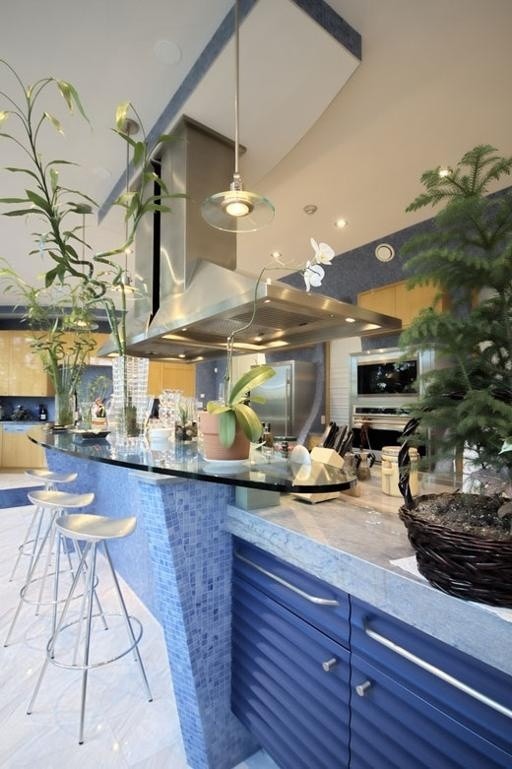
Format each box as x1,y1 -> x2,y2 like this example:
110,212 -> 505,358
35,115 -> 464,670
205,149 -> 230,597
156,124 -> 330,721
208,237 -> 336,448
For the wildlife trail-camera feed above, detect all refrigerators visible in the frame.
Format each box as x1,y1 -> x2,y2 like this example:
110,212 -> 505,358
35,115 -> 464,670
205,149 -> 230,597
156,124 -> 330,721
248,361 -> 318,437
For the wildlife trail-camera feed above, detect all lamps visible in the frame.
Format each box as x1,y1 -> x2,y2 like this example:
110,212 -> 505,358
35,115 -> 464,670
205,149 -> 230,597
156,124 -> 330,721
200,0 -> 276,234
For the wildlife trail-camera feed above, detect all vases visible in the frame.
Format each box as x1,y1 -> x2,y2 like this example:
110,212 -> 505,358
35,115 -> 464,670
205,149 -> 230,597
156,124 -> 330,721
200,413 -> 250,460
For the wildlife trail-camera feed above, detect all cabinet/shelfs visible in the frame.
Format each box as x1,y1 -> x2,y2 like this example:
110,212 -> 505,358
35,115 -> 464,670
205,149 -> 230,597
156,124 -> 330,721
1,328 -> 55,397
357,273 -> 479,340
229,531 -> 512,769
1,422 -> 56,468
58,332 -> 114,367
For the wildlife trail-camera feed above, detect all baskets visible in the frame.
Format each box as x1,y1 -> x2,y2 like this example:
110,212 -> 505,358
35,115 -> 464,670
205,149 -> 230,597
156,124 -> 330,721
398,389 -> 511,607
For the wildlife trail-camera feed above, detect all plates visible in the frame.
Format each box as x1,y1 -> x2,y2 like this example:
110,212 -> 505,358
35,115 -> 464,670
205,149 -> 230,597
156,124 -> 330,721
80,431 -> 110,439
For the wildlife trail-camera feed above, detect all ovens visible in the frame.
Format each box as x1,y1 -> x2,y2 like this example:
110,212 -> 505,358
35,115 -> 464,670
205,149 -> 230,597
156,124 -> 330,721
348,346 -> 433,469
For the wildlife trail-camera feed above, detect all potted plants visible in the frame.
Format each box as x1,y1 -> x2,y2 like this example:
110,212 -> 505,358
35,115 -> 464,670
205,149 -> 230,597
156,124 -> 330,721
0,57 -> 193,458
0,257 -> 98,429
395,133 -> 512,607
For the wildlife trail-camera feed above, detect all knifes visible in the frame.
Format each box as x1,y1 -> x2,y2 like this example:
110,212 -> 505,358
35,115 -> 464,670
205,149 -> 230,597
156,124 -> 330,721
320,421 -> 355,458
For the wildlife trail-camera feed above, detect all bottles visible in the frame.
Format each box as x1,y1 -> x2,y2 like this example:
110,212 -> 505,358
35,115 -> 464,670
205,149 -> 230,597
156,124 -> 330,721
39,404 -> 47,421
379,445 -> 419,497
281,442 -> 290,458
125,390 -> 137,437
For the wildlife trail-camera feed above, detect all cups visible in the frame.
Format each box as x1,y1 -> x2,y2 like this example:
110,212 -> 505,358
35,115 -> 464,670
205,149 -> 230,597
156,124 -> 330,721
262,432 -> 273,447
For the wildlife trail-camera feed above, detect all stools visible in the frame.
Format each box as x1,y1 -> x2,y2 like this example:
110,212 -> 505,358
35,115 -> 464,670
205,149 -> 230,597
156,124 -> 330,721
6,485 -> 105,659
11,467 -> 90,611
24,511 -> 157,741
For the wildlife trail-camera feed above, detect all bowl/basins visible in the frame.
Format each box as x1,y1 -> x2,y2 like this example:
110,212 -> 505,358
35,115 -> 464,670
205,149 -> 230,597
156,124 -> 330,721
151,429 -> 170,441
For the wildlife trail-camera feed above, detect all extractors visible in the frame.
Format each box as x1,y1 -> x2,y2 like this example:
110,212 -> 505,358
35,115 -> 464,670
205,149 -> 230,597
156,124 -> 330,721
94,116 -> 403,363
